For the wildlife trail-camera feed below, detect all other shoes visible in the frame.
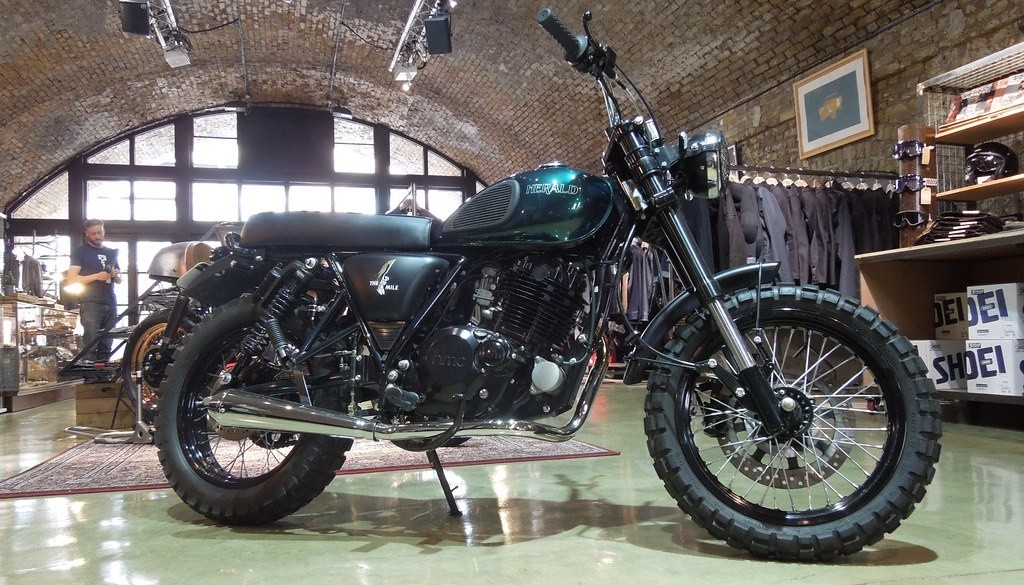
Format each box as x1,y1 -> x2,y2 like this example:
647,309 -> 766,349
99,373 -> 111,383
84,375 -> 99,384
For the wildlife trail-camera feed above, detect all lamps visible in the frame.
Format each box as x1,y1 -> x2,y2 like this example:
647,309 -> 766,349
119,1 -> 150,40
164,49 -> 191,69
424,12 -> 452,56
392,63 -> 418,91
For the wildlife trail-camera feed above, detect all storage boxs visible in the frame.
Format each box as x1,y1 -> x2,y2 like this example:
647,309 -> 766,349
908,284 -> 1024,398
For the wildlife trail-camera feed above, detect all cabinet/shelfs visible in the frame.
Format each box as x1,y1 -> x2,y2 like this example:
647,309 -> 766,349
0,294 -> 85,414
855,42 -> 1024,416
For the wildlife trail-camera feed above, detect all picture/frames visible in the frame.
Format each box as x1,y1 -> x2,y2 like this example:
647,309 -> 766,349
792,49 -> 877,160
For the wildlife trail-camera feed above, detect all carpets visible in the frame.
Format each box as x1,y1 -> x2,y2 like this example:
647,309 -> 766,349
0,433 -> 620,499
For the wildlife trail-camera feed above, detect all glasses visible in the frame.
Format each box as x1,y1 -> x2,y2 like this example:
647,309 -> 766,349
895,174 -> 924,194
893,210 -> 925,228
891,140 -> 923,160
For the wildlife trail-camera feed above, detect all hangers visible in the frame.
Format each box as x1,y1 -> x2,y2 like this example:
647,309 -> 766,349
728,164 -> 897,192
629,237 -> 648,249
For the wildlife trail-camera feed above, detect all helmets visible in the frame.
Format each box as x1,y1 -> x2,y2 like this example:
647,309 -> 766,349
966,141 -> 1018,179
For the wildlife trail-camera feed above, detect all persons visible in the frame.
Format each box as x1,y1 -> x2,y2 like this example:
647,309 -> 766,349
68,219 -> 121,382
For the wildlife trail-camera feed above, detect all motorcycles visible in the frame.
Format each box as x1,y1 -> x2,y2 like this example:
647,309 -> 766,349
158,9 -> 946,569
107,228 -> 242,428
